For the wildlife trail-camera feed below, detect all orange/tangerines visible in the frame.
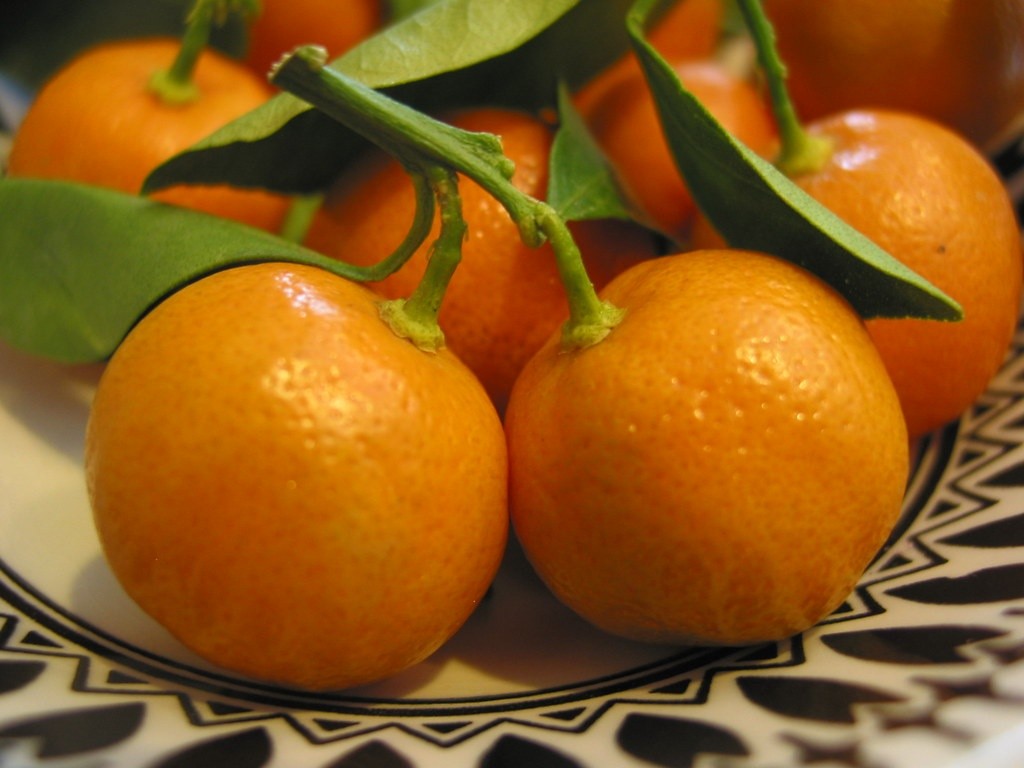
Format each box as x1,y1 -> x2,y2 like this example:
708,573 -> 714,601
0,0 -> 1024,692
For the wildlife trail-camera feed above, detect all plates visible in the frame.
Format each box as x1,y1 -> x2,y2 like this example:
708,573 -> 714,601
0,0 -> 1024,768
